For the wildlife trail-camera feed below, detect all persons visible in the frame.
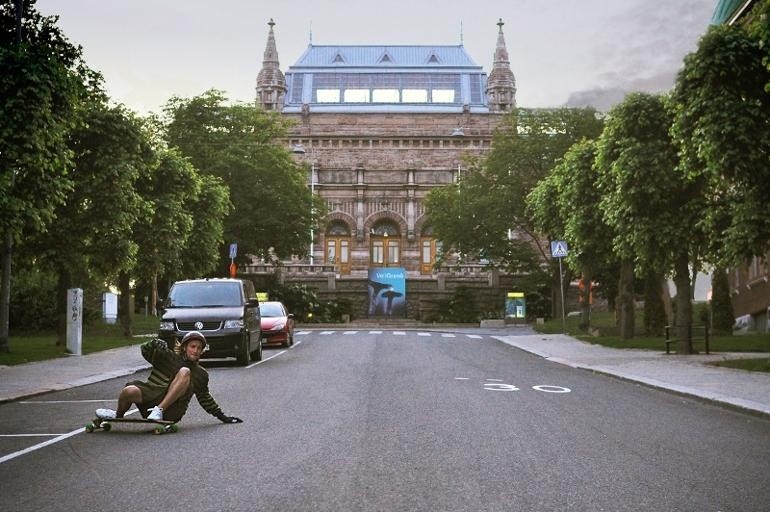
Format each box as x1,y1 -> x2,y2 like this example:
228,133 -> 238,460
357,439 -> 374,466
93,328 -> 244,425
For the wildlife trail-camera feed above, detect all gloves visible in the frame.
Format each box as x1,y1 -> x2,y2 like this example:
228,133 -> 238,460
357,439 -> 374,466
154,339 -> 168,351
219,415 -> 243,424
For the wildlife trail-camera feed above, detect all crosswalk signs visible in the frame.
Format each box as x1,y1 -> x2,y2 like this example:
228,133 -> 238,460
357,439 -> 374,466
551,240 -> 568,257
230,244 -> 237,257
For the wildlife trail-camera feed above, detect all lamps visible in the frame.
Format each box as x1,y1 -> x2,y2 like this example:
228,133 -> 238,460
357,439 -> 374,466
449,126 -> 465,137
294,143 -> 307,154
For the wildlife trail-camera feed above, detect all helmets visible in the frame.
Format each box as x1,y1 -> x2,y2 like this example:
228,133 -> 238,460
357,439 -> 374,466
181,331 -> 206,349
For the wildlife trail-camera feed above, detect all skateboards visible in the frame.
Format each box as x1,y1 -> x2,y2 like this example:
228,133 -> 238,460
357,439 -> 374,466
86,417 -> 178,435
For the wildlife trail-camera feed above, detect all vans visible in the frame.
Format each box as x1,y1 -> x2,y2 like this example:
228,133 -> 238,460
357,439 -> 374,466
157,277 -> 262,366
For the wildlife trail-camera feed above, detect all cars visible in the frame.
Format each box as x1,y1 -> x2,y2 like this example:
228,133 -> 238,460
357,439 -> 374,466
256,293 -> 294,346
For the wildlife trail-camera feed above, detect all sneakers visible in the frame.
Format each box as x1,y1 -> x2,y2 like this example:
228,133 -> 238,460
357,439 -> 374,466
146,406 -> 164,420
96,409 -> 117,419
